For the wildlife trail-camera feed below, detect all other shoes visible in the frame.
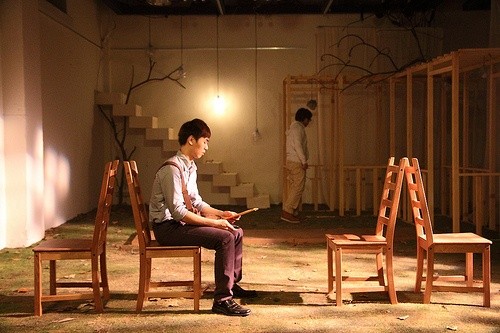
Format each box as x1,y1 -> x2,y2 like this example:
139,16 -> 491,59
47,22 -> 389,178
280,211 -> 301,223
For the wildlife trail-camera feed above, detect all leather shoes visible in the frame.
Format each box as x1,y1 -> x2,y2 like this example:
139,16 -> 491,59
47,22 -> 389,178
212,298 -> 251,315
232,283 -> 256,298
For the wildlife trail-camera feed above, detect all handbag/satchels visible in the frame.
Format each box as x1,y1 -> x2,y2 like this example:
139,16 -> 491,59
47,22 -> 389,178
188,207 -> 201,215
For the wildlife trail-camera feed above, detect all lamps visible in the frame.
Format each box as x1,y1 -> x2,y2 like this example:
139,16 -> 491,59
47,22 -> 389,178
178,17 -> 187,81
145,16 -> 155,57
212,16 -> 225,111
251,15 -> 262,144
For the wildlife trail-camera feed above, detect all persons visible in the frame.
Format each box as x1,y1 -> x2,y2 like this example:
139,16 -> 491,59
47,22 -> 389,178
280,108 -> 313,224
148,118 -> 257,316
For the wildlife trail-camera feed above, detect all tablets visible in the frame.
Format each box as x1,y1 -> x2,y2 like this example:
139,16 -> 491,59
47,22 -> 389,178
223,208 -> 259,220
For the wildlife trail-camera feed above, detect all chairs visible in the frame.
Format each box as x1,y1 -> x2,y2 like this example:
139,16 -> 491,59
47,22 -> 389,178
324,157 -> 407,306
402,157 -> 492,307
124,161 -> 201,313
32,159 -> 119,317
